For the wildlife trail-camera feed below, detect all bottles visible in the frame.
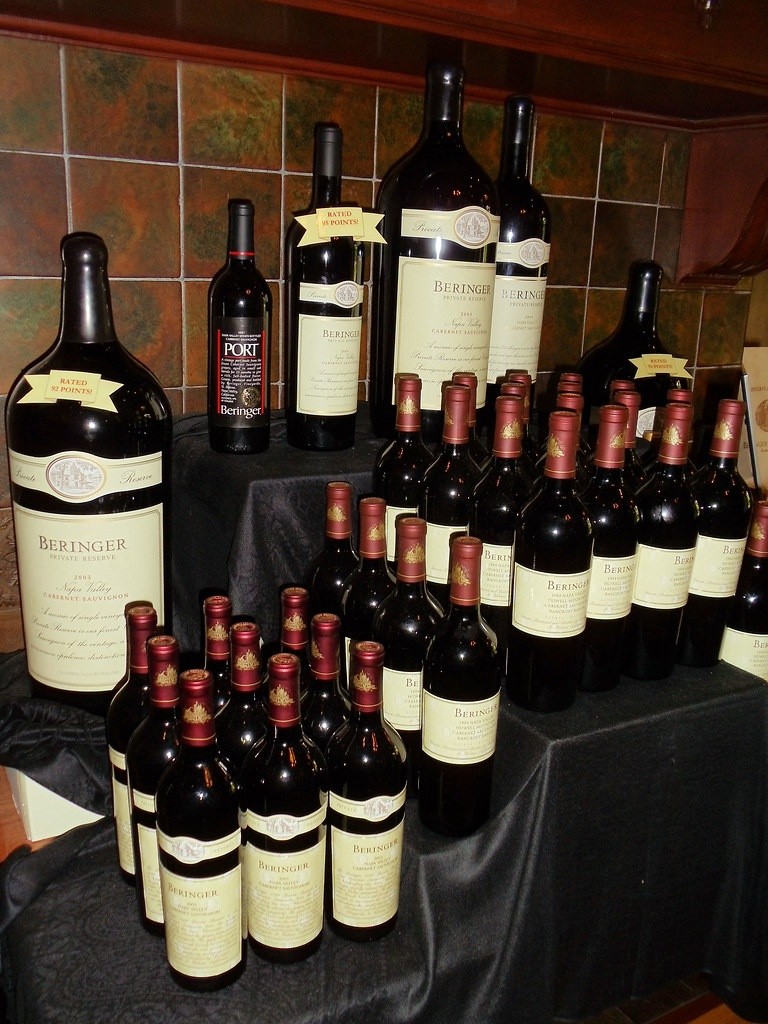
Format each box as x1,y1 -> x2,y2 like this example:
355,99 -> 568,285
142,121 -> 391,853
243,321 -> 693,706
676,399 -> 768,684
528,373 -> 593,497
5,233 -> 175,706
418,537 -> 501,835
270,587 -> 354,760
304,482 -> 359,649
580,405 -> 637,693
370,518 -> 446,799
472,370 -> 539,678
575,259 -> 688,431
421,373 -> 492,618
200,597 -> 270,799
499,411 -> 595,712
373,375 -> 435,574
368,56 -> 501,433
108,605 -> 182,936
588,379 -> 647,496
636,390 -> 698,683
327,642 -> 408,945
155,668 -> 246,992
239,652 -> 328,964
285,123 -> 364,454
483,93 -> 550,414
209,199 -> 272,457
332,498 -> 397,694
